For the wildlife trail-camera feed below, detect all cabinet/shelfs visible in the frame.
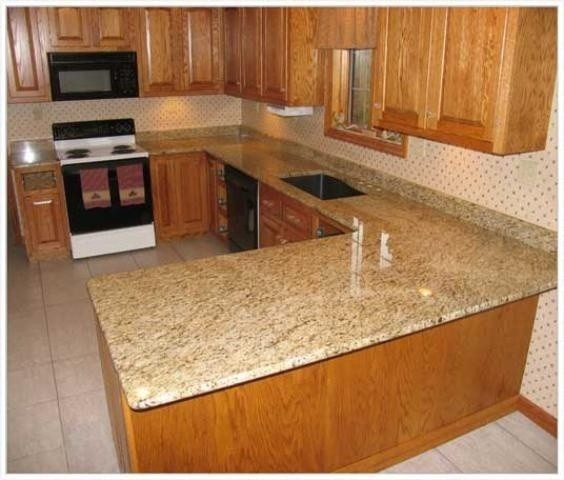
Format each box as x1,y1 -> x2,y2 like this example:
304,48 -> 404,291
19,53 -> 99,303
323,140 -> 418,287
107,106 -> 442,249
224,6 -> 322,106
371,7 -> 557,155
316,7 -> 379,49
209,156 -> 227,243
316,214 -> 353,240
7,7 -> 52,103
42,6 -> 139,52
149,153 -> 209,238
139,6 -> 225,96
11,164 -> 69,265
257,182 -> 315,248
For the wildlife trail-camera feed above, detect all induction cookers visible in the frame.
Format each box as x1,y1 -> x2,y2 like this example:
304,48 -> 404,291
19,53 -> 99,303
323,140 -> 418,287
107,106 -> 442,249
50,134 -> 150,168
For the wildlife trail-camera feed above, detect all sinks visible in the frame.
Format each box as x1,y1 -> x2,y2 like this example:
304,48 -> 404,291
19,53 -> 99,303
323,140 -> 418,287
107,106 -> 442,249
275,168 -> 369,202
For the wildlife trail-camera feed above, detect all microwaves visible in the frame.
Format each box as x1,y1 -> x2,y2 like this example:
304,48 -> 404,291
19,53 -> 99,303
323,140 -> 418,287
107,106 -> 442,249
45,51 -> 139,102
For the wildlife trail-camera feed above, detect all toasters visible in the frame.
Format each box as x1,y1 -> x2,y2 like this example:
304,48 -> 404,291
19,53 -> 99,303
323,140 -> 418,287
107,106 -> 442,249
61,156 -> 154,237
